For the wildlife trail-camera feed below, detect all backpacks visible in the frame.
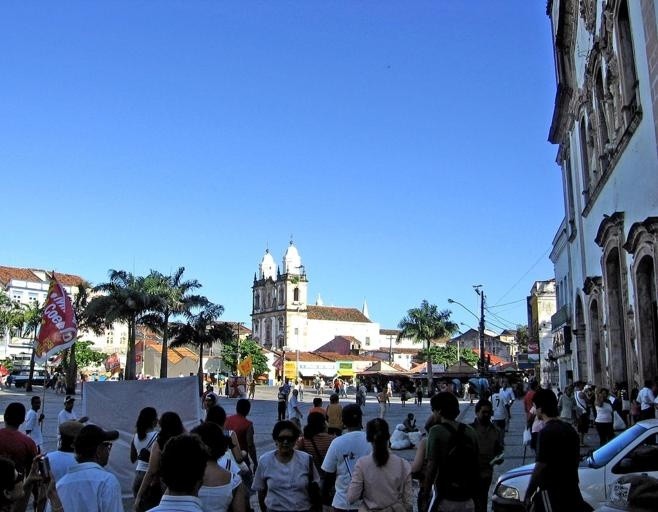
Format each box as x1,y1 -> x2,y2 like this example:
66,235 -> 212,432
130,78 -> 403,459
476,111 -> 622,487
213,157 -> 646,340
433,423 -> 482,500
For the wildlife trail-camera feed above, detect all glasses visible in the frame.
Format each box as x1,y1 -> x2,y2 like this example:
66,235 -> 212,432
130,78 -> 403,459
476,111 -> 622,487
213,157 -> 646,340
277,436 -> 294,443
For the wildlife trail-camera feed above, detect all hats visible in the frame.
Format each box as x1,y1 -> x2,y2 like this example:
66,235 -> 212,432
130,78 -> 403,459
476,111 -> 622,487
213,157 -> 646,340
59,395 -> 119,451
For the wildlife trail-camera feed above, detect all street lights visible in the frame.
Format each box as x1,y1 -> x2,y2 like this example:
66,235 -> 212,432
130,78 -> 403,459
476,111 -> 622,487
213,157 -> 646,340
447,297 -> 481,342
232,320 -> 247,375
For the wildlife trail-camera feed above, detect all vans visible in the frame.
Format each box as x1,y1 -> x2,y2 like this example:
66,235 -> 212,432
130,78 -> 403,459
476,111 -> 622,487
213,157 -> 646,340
5,367 -> 54,388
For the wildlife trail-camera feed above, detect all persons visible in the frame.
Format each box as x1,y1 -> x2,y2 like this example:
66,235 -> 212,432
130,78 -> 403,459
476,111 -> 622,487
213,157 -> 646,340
0,367 -> 657,512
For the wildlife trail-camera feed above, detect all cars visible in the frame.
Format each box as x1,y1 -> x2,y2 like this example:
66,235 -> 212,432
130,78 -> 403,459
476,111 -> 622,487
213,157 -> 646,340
327,377 -> 350,387
491,411 -> 658,511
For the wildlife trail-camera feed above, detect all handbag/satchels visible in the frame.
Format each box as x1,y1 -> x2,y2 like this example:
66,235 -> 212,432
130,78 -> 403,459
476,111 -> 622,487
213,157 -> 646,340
137,448 -> 150,463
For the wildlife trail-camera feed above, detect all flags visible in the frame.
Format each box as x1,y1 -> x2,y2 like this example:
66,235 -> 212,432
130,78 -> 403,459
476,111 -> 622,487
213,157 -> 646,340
235,358 -> 252,377
32,275 -> 77,365
